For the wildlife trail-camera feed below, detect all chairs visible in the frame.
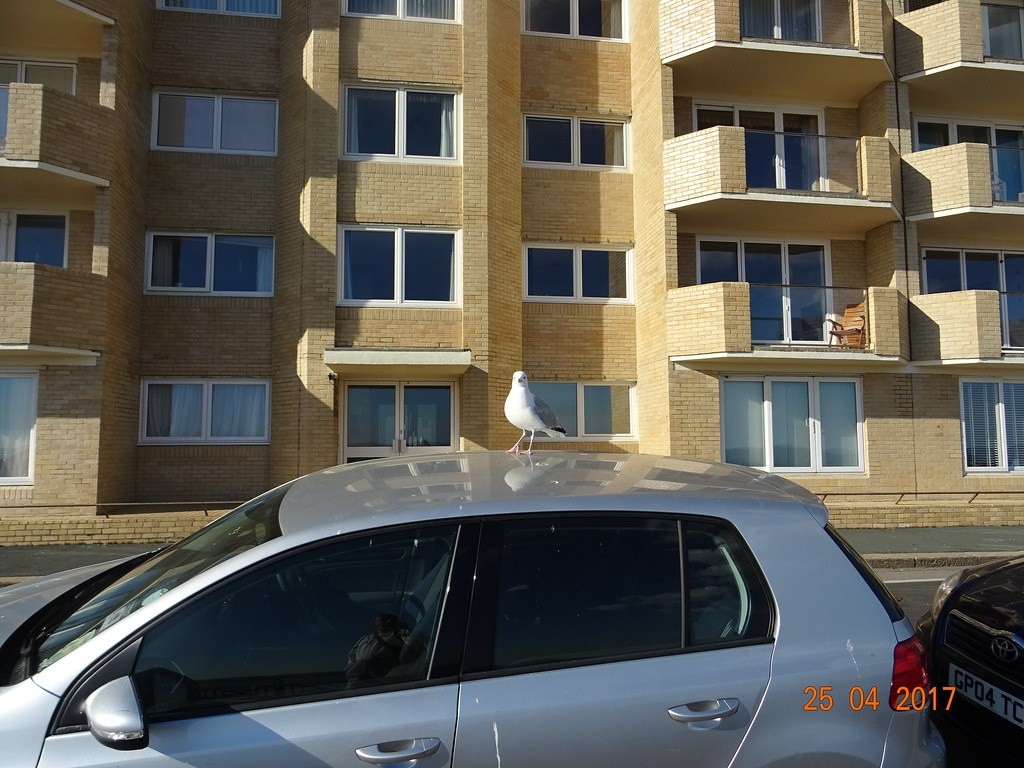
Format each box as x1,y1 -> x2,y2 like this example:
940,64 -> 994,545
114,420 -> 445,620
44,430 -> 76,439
356,538 -> 449,686
826,301 -> 865,349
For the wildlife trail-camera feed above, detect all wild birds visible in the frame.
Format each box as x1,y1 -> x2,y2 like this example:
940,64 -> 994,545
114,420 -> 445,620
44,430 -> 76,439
504,369 -> 568,456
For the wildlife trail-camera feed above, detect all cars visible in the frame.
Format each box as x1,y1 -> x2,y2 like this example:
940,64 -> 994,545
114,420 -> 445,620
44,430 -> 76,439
0,444 -> 946,768
916,553 -> 1024,768
